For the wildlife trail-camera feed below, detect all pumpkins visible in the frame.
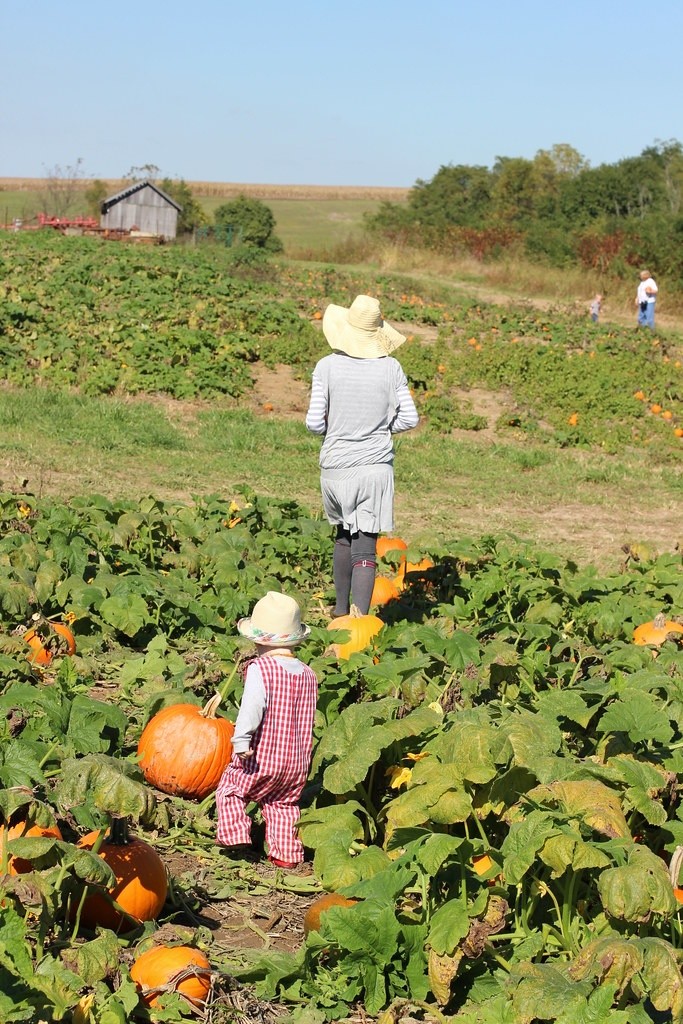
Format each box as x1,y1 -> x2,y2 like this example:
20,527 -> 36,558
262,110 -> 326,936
631,612 -> 683,659
0,536 -> 434,1013
471,854 -> 496,886
667,845 -> 683,905
263,270 -> 683,438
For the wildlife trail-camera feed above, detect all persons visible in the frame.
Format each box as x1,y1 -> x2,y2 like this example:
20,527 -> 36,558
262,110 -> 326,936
635,269 -> 659,331
212,590 -> 317,868
589,294 -> 604,322
304,293 -> 419,620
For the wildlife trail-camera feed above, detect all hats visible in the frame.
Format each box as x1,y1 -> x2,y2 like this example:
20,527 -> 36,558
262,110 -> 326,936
322,295 -> 406,359
238,590 -> 311,647
